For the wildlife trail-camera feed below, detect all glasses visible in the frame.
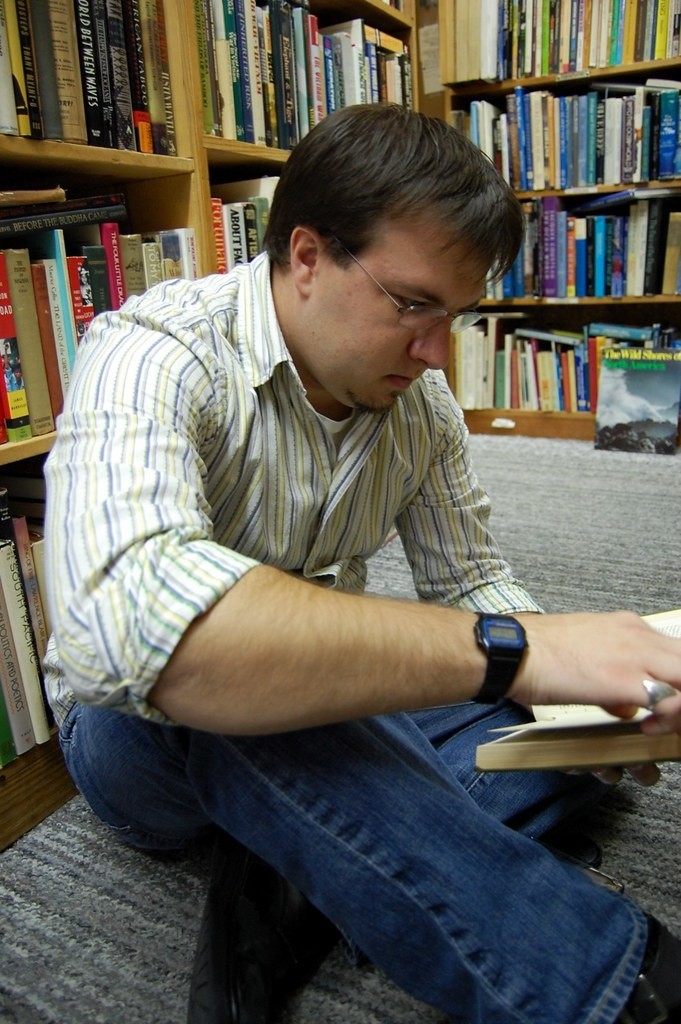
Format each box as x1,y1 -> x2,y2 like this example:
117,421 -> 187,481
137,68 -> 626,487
282,220 -> 482,334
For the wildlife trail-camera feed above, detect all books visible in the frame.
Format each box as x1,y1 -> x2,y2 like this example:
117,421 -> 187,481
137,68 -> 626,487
193,0 -> 413,153
451,0 -> 681,410
0,476 -> 59,770
0,187 -> 198,444
473,609 -> 681,772
210,196 -> 270,275
0,0 -> 178,157
594,346 -> 681,456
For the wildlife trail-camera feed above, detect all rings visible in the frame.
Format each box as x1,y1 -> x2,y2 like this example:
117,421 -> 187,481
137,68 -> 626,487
642,679 -> 676,711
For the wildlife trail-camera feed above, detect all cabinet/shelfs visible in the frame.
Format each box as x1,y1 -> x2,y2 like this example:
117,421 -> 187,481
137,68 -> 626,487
0,0 -> 681,853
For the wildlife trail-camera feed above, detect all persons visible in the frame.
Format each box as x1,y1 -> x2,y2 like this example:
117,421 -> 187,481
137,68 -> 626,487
41,102 -> 681,1024
4,343 -> 25,391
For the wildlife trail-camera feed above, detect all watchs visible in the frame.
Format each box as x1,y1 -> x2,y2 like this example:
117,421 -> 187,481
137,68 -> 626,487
471,612 -> 529,705
